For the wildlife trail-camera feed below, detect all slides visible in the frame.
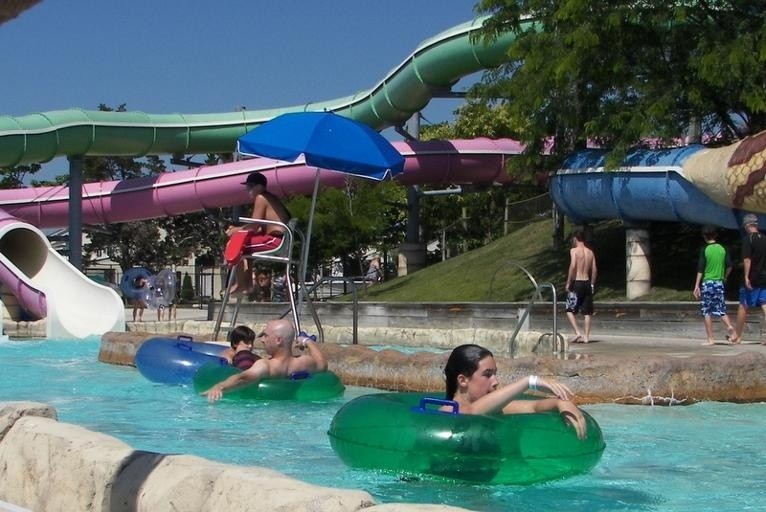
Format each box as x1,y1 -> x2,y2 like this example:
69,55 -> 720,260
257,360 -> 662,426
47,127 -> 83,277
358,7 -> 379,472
549,107 -> 766,234
1,13 -> 556,176
1,131 -> 734,338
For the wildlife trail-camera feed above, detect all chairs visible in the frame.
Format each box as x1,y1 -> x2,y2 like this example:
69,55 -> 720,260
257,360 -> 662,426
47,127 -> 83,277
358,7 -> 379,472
247,217 -> 301,259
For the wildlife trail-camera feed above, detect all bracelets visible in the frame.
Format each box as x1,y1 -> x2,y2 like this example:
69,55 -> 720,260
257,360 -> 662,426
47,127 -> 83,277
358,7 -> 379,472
302,337 -> 309,347
528,374 -> 537,390
590,284 -> 594,287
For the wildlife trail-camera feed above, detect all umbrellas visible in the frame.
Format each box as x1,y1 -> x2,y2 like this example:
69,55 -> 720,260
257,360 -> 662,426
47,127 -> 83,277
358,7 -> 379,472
235,109 -> 406,316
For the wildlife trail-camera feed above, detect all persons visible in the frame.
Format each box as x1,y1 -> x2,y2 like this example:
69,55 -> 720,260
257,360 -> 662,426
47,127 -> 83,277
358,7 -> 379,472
248,267 -> 295,302
437,343 -> 588,440
218,172 -> 291,297
365,254 -> 384,287
725,212 -> 766,345
132,274 -> 176,321
198,319 -> 328,404
217,325 -> 256,365
563,230 -> 598,343
693,224 -> 740,347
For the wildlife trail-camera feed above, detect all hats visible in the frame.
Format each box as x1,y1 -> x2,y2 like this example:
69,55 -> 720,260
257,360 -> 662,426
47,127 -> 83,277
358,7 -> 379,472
239,171 -> 268,187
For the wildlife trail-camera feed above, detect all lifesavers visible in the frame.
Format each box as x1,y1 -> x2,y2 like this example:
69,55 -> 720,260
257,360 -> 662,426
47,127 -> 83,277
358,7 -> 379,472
326,392 -> 606,485
135,335 -> 229,387
193,359 -> 346,404
